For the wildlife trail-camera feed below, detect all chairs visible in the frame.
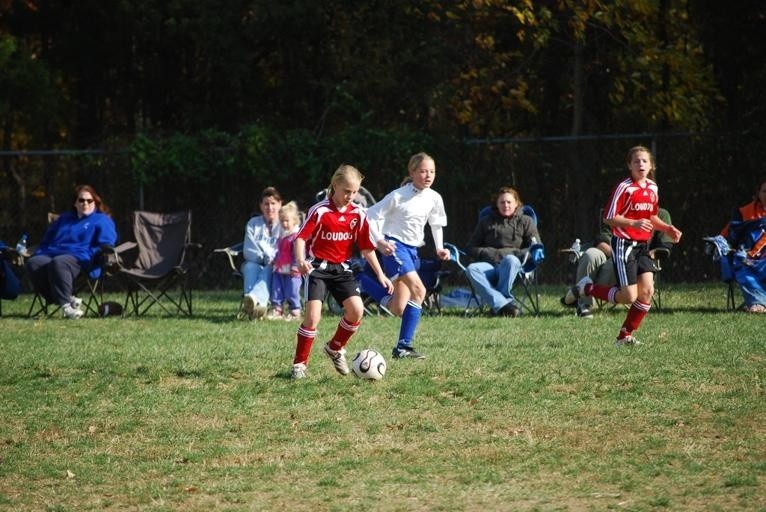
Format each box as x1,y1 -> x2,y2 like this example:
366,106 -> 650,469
704,227 -> 764,316
102,211 -> 200,316
226,243 -> 314,321
460,206 -> 542,319
23,220 -> 101,320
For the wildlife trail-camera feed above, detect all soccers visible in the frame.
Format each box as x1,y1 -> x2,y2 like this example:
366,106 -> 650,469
351,349 -> 387,381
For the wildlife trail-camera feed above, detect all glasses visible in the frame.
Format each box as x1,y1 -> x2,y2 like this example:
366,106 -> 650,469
78,198 -> 94,203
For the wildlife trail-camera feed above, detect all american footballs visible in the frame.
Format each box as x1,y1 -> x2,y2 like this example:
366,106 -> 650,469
98,302 -> 123,316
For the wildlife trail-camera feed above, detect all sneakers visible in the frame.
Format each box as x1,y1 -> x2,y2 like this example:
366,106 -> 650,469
490,302 -> 519,317
291,364 -> 306,378
617,334 -> 642,344
392,346 -> 424,358
325,341 -> 349,375
745,304 -> 766,313
561,276 -> 593,318
350,261 -> 364,273
243,293 -> 301,321
63,295 -> 84,318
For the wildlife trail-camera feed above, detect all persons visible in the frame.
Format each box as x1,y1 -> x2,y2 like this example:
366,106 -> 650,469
564,145 -> 683,347
365,151 -> 451,361
25,185 -> 117,319
289,164 -> 395,380
726,181 -> 766,315
240,188 -> 398,320
464,186 -> 542,318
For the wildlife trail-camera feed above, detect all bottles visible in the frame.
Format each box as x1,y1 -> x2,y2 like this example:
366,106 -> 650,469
567,238 -> 581,264
11,233 -> 28,266
